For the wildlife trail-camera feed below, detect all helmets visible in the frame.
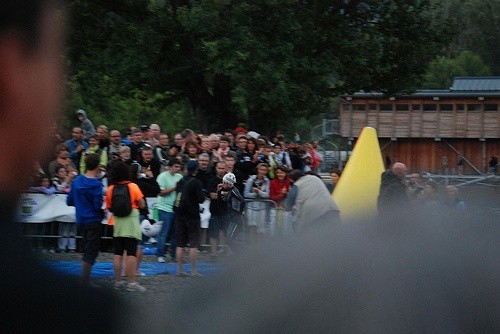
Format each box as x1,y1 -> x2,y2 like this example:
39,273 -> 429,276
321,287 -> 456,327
222,172 -> 236,184
140,219 -> 164,236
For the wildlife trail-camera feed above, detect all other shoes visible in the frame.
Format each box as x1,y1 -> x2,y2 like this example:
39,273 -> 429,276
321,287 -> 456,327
176,270 -> 190,277
158,256 -> 166,262
191,272 -> 204,278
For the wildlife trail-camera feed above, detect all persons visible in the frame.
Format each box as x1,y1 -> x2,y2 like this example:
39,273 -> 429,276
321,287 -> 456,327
157,159 -> 184,262
284,169 -> 342,239
487,157 -> 500,176
173,161 -> 205,278
217,172 -> 246,251
0,0 -> 139,334
406,172 -> 465,216
66,153 -> 104,278
328,170 -> 341,193
106,161 -> 147,293
25,109 -> 323,256
377,163 -> 407,217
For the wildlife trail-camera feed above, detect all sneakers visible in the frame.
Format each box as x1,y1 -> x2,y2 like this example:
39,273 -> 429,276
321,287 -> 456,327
114,280 -> 128,290
126,282 -> 146,292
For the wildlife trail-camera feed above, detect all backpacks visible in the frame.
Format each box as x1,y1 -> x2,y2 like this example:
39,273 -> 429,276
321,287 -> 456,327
110,182 -> 132,217
